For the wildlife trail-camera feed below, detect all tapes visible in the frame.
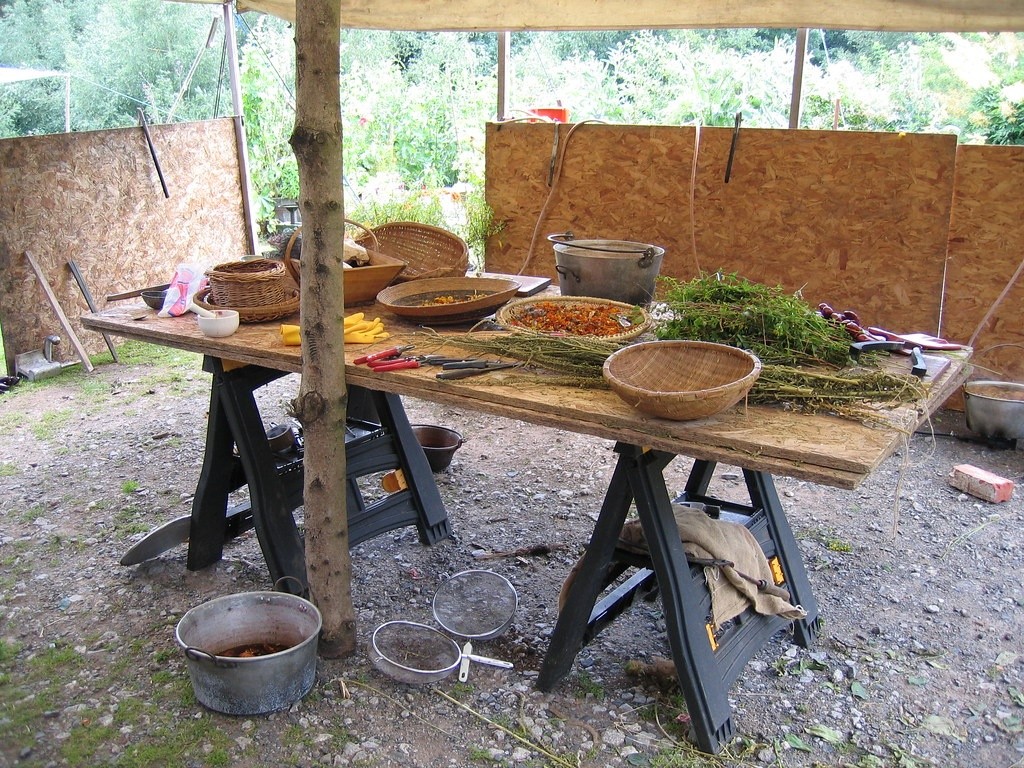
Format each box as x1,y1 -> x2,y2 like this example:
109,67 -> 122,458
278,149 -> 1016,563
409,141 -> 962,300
265,423 -> 296,452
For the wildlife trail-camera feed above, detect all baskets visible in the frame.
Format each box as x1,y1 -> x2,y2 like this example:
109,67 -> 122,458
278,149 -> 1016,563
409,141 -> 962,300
194,258 -> 301,324
376,277 -> 522,324
603,339 -> 761,421
262,218 -> 408,307
496,295 -> 652,342
355,221 -> 470,285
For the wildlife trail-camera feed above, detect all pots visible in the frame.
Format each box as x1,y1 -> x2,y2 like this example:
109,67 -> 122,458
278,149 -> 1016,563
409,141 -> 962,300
177,578 -> 324,716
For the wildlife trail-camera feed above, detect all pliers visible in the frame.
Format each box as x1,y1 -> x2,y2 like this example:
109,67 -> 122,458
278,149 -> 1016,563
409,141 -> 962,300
366,353 -> 446,373
352,342 -> 416,365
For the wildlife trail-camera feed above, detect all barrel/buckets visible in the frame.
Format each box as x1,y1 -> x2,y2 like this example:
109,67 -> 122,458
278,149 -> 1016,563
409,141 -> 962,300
962,344 -> 1024,441
547,231 -> 665,309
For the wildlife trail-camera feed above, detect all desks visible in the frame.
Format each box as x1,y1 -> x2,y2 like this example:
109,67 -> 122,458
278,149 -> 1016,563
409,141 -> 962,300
79,269 -> 975,756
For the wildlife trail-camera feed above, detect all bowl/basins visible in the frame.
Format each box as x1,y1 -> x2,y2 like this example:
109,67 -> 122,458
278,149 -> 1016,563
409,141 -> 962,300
409,423 -> 464,473
197,310 -> 240,337
141,291 -> 168,310
240,255 -> 263,261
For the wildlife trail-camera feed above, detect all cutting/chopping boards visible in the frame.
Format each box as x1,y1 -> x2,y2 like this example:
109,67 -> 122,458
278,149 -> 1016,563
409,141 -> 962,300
464,272 -> 552,296
